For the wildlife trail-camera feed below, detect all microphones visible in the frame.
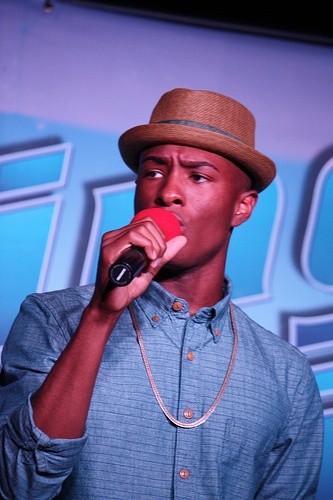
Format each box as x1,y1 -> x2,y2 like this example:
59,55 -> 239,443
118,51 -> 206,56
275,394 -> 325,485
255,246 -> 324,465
107,207 -> 182,286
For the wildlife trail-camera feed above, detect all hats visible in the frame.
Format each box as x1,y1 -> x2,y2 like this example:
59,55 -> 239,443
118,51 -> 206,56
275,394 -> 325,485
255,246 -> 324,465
118,86 -> 276,194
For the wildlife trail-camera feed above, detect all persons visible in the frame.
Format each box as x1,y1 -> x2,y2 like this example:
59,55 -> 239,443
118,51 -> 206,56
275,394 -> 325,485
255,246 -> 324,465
1,86 -> 326,499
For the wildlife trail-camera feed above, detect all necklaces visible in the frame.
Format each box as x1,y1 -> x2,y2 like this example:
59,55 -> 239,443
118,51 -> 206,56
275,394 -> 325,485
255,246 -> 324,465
116,300 -> 240,430
